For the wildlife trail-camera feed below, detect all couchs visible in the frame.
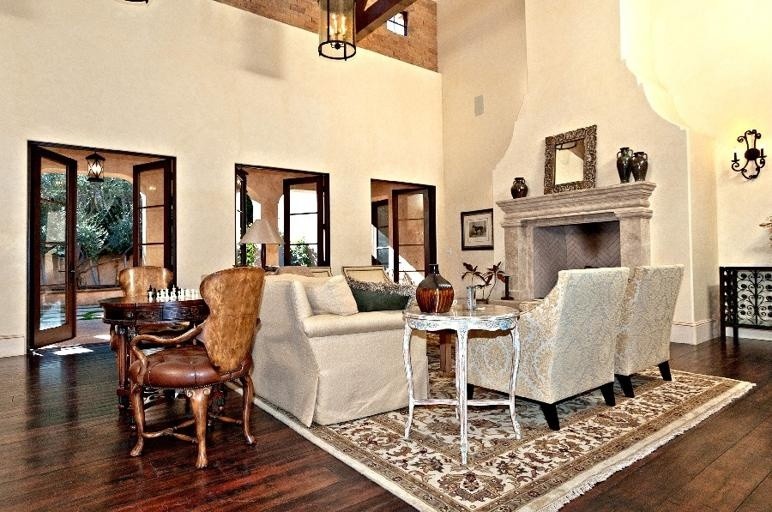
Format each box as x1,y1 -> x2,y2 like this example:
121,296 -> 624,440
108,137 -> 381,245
250,274 -> 429,427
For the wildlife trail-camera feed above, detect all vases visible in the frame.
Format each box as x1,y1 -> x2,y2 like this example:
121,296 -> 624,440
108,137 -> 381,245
627,151 -> 648,181
511,176 -> 528,199
416,264 -> 454,313
616,147 -> 633,184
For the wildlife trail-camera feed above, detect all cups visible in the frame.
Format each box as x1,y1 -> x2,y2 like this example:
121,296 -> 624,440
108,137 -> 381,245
466,286 -> 476,312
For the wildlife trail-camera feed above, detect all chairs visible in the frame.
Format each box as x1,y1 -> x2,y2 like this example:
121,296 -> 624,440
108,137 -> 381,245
97,265 -> 266,469
615,264 -> 684,398
467,267 -> 630,430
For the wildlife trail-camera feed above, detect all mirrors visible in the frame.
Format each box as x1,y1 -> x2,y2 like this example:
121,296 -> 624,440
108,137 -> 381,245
544,125 -> 596,195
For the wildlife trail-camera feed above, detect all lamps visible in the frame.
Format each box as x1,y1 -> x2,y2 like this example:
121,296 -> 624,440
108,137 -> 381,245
240,220 -> 281,269
731,130 -> 767,180
318,0 -> 356,60
85,151 -> 105,182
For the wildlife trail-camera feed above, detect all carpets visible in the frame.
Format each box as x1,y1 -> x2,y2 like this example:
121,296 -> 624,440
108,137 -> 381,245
223,332 -> 758,512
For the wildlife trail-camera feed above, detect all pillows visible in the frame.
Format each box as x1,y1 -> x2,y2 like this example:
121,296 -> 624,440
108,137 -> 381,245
284,275 -> 358,316
346,276 -> 414,312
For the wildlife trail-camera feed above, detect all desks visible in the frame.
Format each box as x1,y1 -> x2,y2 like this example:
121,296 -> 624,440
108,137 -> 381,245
402,304 -> 521,465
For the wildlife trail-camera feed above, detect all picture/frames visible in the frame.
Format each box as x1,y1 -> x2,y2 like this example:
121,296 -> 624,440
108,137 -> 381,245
461,208 -> 493,250
